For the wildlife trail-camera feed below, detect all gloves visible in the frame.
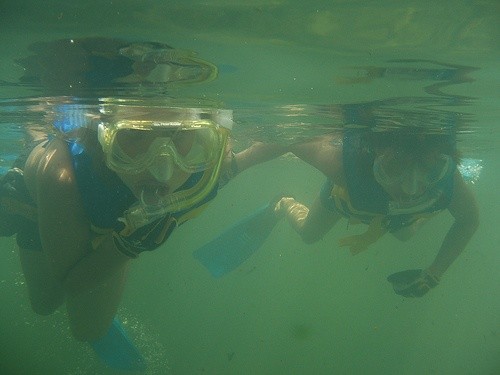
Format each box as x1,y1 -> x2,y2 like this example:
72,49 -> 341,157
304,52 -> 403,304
113,201 -> 174,255
387,269 -> 436,297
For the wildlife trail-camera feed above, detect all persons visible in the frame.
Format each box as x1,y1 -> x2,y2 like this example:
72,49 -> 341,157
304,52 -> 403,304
2,103 -> 300,374
191,126 -> 481,299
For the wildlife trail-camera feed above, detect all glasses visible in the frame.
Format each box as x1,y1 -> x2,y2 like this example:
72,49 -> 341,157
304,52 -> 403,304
97,118 -> 219,174
